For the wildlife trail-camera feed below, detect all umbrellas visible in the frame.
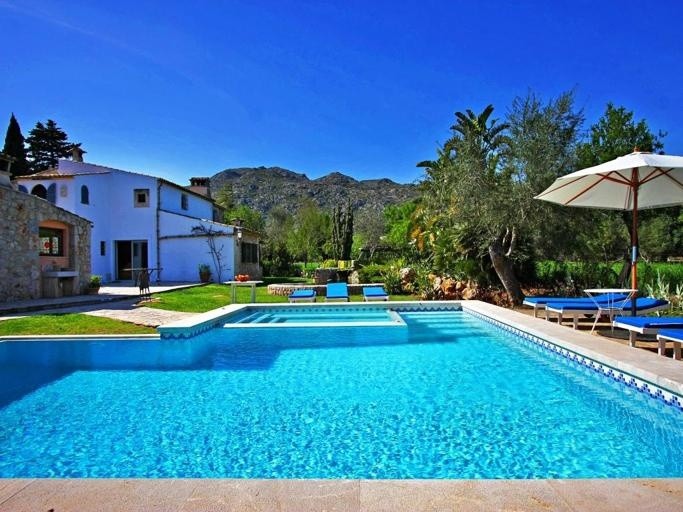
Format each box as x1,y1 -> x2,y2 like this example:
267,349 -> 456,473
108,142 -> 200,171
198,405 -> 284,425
531,143 -> 683,315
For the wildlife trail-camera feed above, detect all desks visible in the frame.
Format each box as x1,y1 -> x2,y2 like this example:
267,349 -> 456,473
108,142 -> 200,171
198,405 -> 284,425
223,281 -> 264,303
584,289 -> 639,337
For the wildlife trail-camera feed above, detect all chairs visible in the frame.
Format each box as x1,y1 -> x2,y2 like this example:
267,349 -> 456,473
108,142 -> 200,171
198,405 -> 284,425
522,294 -> 683,360
288,282 -> 389,303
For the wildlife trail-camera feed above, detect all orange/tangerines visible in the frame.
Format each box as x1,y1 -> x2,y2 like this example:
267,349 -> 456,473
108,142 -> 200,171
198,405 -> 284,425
234,274 -> 250,280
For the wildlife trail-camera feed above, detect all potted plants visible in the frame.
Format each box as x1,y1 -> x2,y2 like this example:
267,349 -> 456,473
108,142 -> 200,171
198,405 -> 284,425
198,263 -> 214,282
88,275 -> 102,294
